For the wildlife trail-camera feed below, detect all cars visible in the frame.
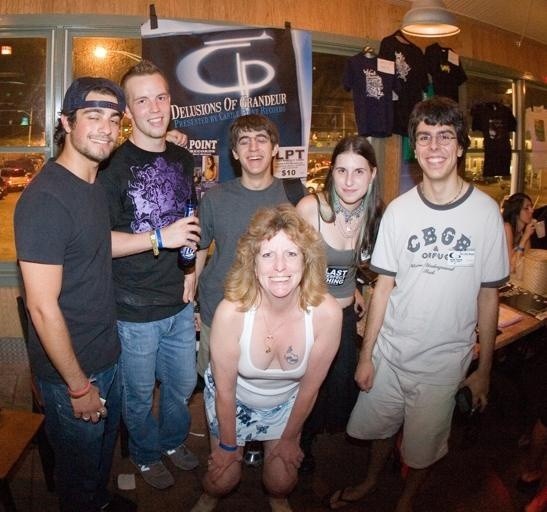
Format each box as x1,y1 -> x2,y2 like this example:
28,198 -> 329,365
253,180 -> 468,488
474,173 -> 500,185
306,178 -> 326,193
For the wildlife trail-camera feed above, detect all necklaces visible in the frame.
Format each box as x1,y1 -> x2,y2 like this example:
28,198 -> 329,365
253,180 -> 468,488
421,182 -> 464,206
262,297 -> 292,353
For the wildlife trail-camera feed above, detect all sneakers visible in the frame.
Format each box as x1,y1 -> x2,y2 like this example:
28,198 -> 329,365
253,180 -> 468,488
129,456 -> 175,490
162,443 -> 200,472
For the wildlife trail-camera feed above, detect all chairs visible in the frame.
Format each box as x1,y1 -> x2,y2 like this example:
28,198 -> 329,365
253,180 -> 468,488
16,295 -> 131,493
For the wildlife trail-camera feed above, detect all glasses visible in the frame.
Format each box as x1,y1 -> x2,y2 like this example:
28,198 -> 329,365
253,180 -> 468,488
414,133 -> 460,147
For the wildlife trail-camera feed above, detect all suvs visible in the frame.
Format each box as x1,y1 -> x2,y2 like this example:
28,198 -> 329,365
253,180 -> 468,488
1,167 -> 28,192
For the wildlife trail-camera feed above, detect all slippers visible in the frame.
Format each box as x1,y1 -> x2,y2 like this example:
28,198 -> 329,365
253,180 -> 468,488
324,485 -> 365,511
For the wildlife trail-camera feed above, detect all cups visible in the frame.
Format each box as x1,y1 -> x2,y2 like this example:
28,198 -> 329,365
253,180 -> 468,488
535,220 -> 546,239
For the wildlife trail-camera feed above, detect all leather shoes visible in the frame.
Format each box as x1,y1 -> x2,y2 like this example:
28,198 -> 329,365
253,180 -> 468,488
95,494 -> 137,512
299,442 -> 316,475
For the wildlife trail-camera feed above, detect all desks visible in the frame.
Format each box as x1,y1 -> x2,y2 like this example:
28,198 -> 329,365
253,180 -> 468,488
475,283 -> 547,360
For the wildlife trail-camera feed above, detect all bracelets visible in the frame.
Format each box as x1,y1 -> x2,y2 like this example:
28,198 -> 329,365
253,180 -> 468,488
68,384 -> 91,399
150,231 -> 160,256
517,247 -> 525,251
156,229 -> 162,249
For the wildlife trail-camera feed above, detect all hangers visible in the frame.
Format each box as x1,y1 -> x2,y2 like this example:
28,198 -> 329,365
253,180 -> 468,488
391,26 -> 412,45
357,44 -> 380,57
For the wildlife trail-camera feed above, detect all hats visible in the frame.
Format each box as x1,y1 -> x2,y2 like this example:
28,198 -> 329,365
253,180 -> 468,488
62,74 -> 128,117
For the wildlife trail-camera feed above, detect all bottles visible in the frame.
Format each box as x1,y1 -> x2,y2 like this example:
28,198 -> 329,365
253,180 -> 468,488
178,198 -> 199,269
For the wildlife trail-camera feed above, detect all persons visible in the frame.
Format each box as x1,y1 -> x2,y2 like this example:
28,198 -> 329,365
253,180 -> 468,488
192,205 -> 343,512
97,62 -> 202,491
322,97 -> 510,511
13,76 -> 127,510
502,192 -> 536,274
195,114 -> 311,378
295,137 -> 387,473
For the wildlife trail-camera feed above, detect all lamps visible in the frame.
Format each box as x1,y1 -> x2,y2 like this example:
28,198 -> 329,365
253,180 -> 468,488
400,1 -> 464,39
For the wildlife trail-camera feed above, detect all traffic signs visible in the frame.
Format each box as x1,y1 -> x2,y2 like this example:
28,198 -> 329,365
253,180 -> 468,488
94,46 -> 142,64
18,106 -> 34,145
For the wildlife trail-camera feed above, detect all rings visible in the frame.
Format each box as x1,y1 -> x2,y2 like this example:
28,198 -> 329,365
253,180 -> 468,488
82,416 -> 91,421
